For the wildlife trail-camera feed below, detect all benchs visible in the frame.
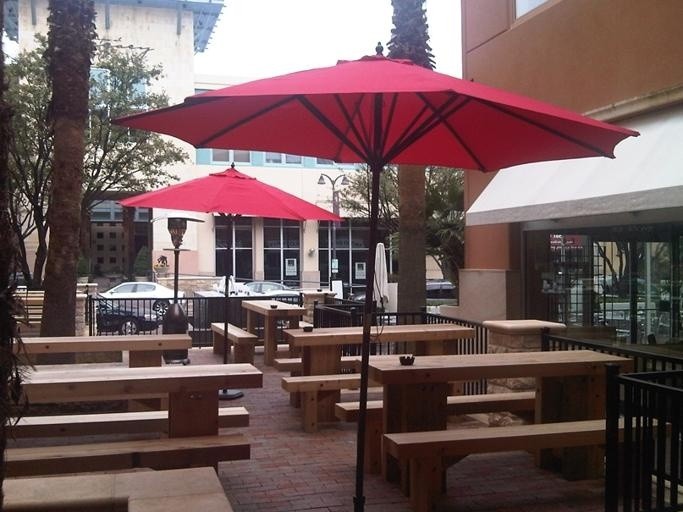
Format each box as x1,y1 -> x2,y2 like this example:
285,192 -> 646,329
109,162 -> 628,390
0,284 -> 262,512
274,320 -> 673,510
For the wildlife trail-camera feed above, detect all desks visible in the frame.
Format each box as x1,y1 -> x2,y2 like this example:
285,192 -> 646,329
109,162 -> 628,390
241,300 -> 307,366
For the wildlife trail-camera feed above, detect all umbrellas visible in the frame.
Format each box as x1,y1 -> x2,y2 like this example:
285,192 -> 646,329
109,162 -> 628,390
114,163 -> 345,393
107,42 -> 640,512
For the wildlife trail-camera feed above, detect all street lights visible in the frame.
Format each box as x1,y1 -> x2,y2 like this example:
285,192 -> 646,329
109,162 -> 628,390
317,173 -> 349,293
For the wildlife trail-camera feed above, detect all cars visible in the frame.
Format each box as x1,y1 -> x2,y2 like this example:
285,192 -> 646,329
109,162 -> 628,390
94,292 -> 159,335
4,273 -> 43,290
238,281 -> 304,325
552,272 -> 682,313
97,280 -> 187,316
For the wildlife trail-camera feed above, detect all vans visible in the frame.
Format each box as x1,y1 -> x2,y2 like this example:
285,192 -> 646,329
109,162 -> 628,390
351,281 -> 458,313
